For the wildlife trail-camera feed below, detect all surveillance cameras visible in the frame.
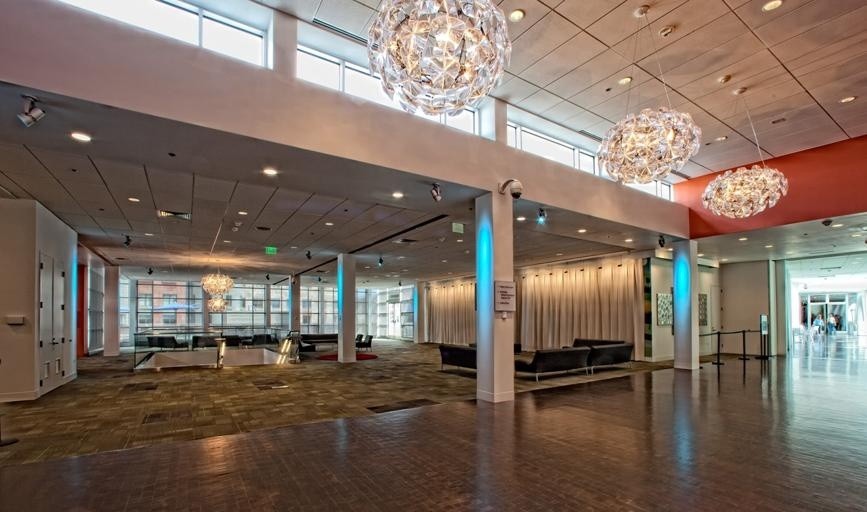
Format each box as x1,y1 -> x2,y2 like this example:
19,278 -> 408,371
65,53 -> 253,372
510,180 -> 523,200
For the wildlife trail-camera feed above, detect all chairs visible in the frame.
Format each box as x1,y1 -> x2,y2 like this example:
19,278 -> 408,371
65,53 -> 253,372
356,335 -> 373,353
354,334 -> 364,344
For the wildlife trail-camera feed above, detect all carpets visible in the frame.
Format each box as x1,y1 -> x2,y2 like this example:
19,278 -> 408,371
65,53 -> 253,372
321,353 -> 378,360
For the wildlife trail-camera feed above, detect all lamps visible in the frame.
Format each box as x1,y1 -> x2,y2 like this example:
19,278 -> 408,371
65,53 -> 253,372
265,274 -> 270,280
698,87 -> 790,222
123,235 -> 131,248
17,94 -> 45,127
367,1 -> 512,117
398,281 -> 401,286
497,179 -> 521,201
658,235 -> 665,248
206,295 -> 227,313
431,183 -> 442,202
198,258 -> 235,295
377,257 -> 383,266
600,5 -> 701,186
318,274 -> 322,281
147,267 -> 153,275
535,208 -> 547,222
306,250 -> 311,259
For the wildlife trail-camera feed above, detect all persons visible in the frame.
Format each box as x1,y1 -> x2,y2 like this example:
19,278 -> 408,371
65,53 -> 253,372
811,311 -> 844,335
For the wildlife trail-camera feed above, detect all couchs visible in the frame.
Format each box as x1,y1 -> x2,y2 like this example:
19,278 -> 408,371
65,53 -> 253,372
514,346 -> 589,383
589,341 -> 633,370
148,332 -> 337,352
438,345 -> 476,374
563,338 -> 625,369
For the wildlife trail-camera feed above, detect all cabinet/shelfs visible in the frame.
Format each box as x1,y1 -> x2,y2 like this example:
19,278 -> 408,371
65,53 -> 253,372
376,285 -> 413,340
